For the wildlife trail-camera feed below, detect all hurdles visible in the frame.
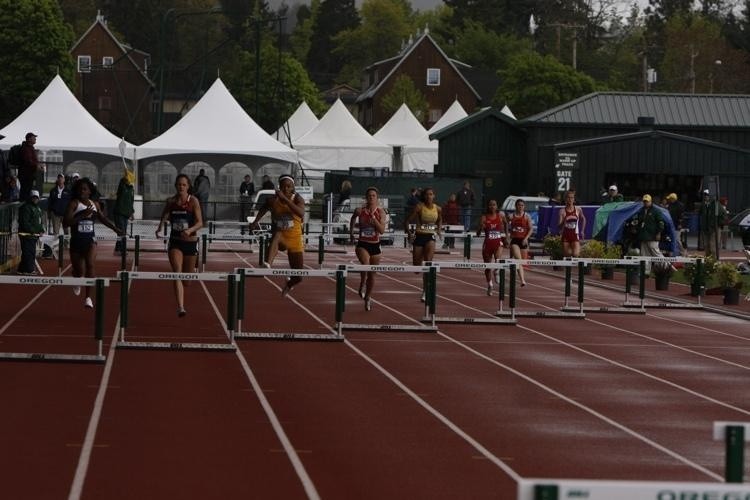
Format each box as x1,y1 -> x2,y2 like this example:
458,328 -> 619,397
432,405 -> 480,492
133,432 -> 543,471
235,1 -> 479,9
517,422 -> 750,500
464,231 -> 511,258
620,255 -> 705,309
202,236 -> 263,267
57,233 -> 129,282
0,276 -> 109,362
233,267 -> 347,343
559,257 -> 646,313
334,264 -> 439,334
115,270 -> 238,354
125,220 -> 466,253
411,230 -> 472,263
420,260 -> 520,324
135,234 -> 201,274
265,233 -> 323,268
494,258 -> 587,317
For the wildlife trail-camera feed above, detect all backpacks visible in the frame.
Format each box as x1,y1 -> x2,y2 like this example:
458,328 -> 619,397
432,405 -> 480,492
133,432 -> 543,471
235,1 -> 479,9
9,145 -> 24,166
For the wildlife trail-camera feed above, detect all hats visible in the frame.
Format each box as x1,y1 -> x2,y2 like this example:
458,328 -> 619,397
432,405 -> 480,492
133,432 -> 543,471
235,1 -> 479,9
0,135 -> 5,140
30,190 -> 39,198
26,133 -> 37,138
667,193 -> 676,199
609,185 -> 617,190
72,173 -> 79,178
57,173 -> 64,178
643,195 -> 652,201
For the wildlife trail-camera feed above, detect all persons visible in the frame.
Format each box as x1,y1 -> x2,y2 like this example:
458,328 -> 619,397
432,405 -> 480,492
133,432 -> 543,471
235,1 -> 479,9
249,174 -> 305,298
155,173 -> 203,317
0,132 -> 99,277
62,179 -> 124,309
538,184 -> 731,277
112,173 -> 135,255
477,198 -> 509,296
239,174 -> 254,196
338,181 -> 352,204
262,175 -> 275,190
405,188 -> 442,302
405,179 -> 477,249
193,168 -> 210,221
350,186 -> 386,311
506,199 -> 533,287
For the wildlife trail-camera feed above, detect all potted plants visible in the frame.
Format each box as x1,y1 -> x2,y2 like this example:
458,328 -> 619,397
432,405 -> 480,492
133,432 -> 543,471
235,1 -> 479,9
541,232 -> 739,306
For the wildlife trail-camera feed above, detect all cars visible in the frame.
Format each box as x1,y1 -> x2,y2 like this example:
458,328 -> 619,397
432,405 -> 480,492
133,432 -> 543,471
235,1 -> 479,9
332,199 -> 395,247
501,196 -> 553,238
246,189 -> 310,247
